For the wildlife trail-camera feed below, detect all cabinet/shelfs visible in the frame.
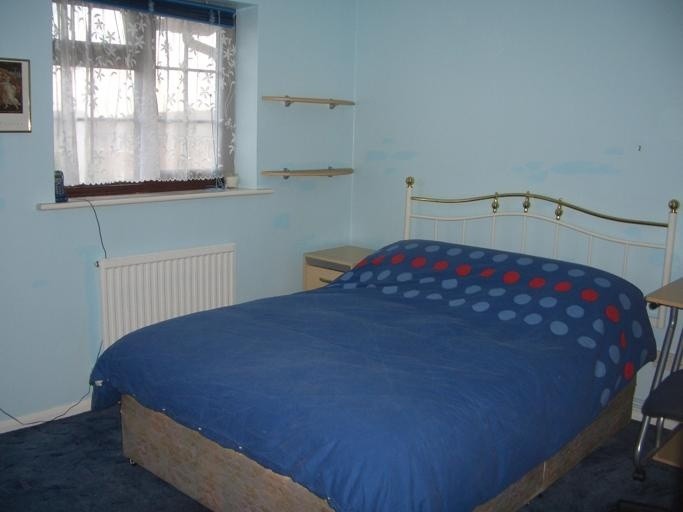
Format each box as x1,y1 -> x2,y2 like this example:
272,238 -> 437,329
261,96 -> 355,181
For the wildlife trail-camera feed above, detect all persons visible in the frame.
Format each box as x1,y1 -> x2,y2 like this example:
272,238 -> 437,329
0,67 -> 20,111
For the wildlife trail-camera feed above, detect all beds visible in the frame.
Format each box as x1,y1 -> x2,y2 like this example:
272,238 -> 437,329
114,172 -> 679,512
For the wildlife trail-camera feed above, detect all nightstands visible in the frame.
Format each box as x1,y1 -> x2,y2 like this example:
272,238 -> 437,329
301,245 -> 378,292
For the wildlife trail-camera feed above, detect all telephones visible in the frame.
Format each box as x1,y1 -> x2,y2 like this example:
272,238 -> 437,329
54,170 -> 68,203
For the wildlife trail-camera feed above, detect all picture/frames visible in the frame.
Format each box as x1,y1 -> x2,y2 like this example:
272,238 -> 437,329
0,57 -> 32,134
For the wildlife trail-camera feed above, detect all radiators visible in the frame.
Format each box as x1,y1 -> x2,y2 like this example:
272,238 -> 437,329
95,242 -> 236,351
